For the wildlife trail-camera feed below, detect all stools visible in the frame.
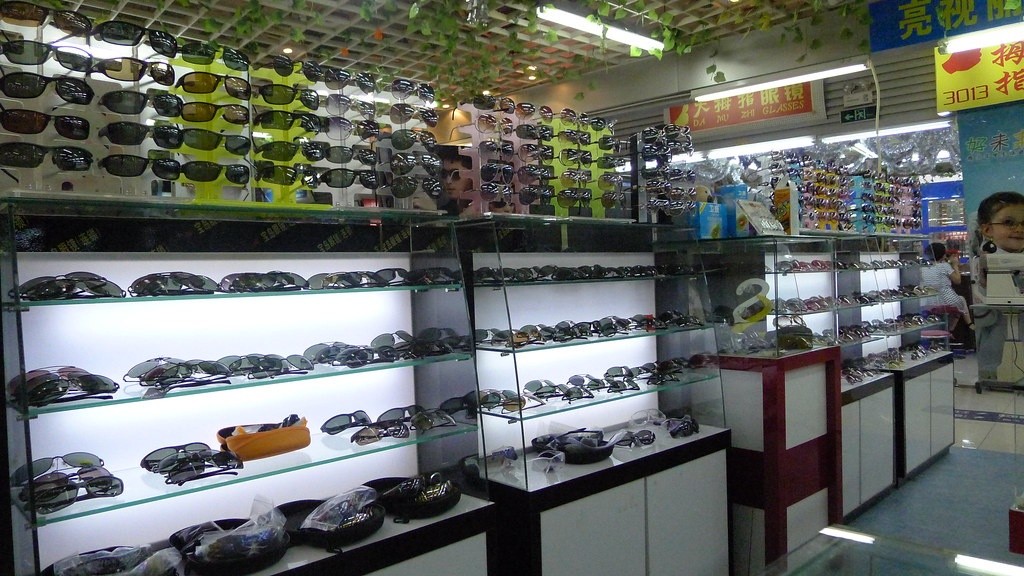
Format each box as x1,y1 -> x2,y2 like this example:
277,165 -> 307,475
932,307 -> 961,351
920,330 -> 953,351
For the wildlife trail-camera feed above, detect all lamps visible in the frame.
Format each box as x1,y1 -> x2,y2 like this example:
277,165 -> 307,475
534,0 -> 665,50
691,56 -> 872,102
936,21 -> 1024,55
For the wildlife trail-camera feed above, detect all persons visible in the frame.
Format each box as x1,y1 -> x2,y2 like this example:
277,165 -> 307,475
489,203 -> 516,212
918,242 -> 976,339
673,280 -> 747,427
438,150 -> 471,217
978,192 -> 1024,381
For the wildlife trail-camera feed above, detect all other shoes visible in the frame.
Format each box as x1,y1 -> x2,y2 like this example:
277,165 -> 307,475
966,322 -> 975,333
948,330 -> 956,342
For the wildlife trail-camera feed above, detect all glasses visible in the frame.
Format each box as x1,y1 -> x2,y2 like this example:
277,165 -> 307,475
988,219 -> 1024,231
0,0 -> 953,576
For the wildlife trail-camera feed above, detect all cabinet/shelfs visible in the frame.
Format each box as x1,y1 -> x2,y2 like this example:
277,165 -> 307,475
0,23 -> 957,576
